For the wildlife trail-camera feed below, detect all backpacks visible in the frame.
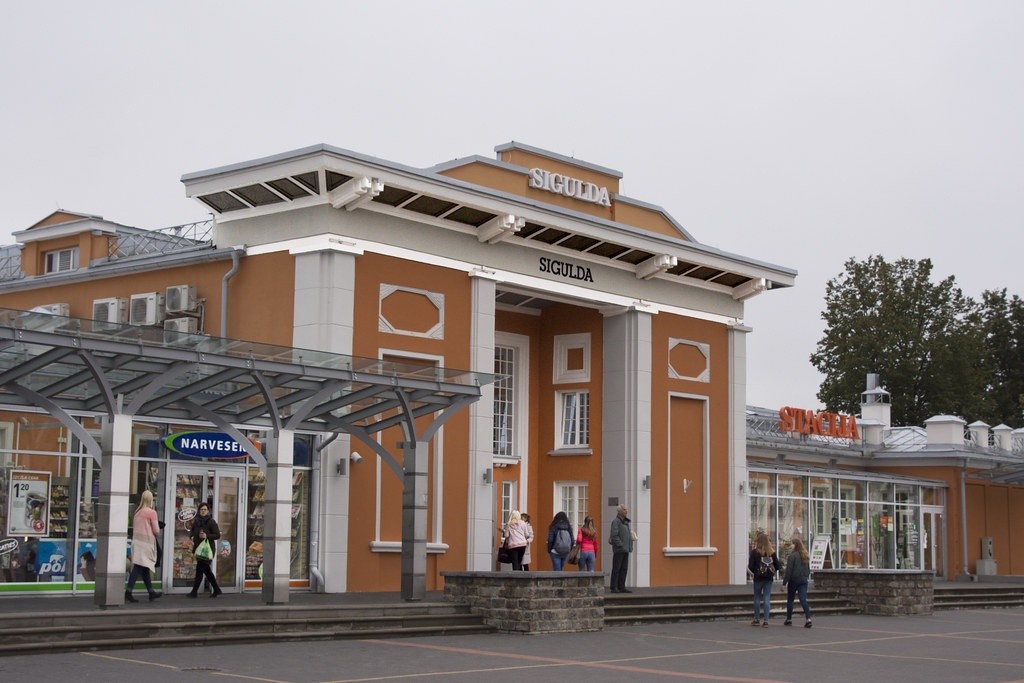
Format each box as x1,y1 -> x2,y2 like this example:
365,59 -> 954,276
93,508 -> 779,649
553,529 -> 571,554
755,552 -> 775,577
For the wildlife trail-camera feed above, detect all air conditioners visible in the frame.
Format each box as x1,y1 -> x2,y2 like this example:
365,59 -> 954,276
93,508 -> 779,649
163,317 -> 198,345
167,285 -> 197,314
92,297 -> 129,331
130,292 -> 167,325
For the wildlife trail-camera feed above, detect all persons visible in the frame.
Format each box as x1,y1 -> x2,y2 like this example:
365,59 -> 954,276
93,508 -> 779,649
782,538 -> 813,629
575,516 -> 598,571
610,504 -> 634,593
548,512 -> 575,571
504,510 -> 529,571
521,513 -> 534,571
186,503 -> 223,598
748,534 -> 782,627
125,490 -> 162,603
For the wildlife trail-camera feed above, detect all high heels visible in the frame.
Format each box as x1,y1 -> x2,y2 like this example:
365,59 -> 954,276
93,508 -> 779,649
125,590 -> 139,602
149,591 -> 162,601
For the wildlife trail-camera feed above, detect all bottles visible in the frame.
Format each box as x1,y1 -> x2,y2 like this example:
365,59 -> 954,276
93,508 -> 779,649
49,485 -> 69,537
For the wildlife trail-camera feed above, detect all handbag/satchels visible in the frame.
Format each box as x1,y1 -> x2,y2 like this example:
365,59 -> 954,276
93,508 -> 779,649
193,538 -> 212,561
521,540 -> 531,565
497,537 -> 512,563
629,530 -> 638,541
568,544 -> 581,564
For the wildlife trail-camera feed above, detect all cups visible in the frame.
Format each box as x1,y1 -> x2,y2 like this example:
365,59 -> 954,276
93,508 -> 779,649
23,491 -> 48,529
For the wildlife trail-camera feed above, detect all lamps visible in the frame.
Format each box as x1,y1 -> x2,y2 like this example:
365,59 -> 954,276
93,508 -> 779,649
336,451 -> 363,476
642,475 -> 651,489
482,467 -> 492,483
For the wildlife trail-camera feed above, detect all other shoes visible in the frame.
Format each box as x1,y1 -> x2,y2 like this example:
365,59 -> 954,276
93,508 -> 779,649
620,588 -> 631,593
762,622 -> 768,627
186,591 -> 196,598
210,589 -> 222,598
611,588 -> 620,593
784,620 -> 792,626
751,620 -> 760,626
804,621 -> 812,627
